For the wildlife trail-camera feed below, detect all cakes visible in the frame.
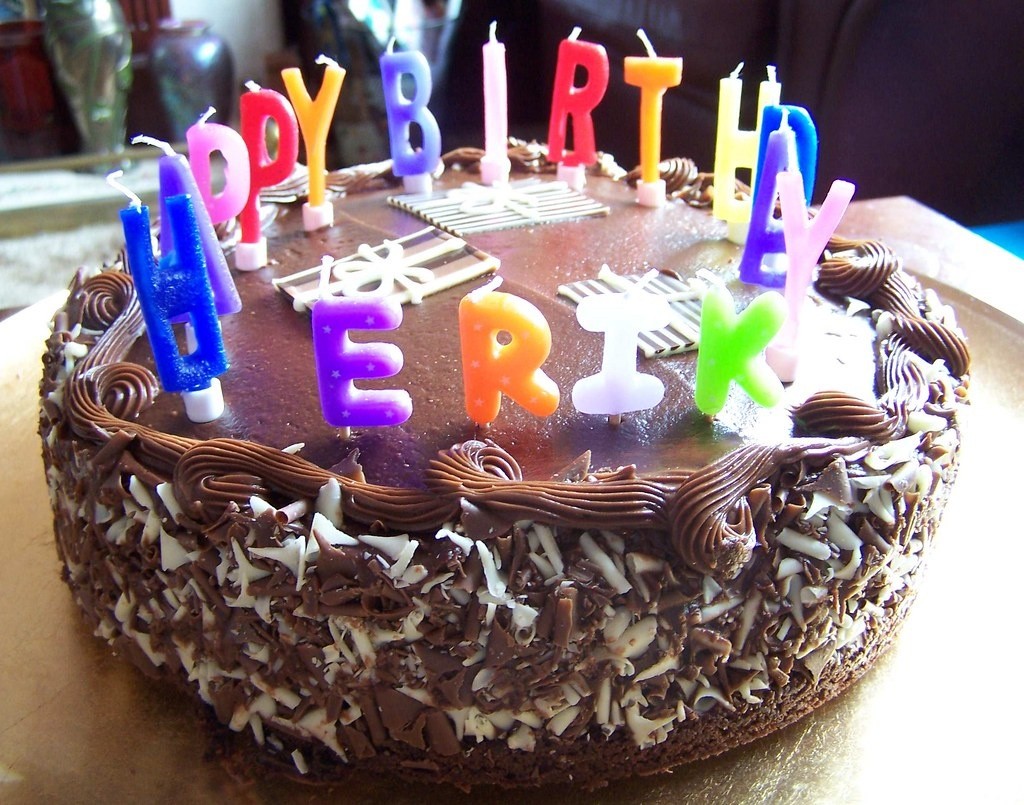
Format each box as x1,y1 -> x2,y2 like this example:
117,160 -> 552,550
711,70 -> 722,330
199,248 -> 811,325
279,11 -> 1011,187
40,140 -> 969,794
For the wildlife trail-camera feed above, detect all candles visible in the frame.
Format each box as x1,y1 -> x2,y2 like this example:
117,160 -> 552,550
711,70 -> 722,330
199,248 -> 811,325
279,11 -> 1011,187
106,22 -> 859,425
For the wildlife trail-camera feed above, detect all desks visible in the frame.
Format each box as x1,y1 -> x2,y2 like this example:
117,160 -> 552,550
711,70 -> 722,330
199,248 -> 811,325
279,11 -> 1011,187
0,197 -> 1024,326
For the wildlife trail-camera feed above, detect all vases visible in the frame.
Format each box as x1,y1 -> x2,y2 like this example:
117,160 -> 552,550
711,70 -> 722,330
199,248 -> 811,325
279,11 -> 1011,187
150,19 -> 235,141
39,0 -> 134,175
